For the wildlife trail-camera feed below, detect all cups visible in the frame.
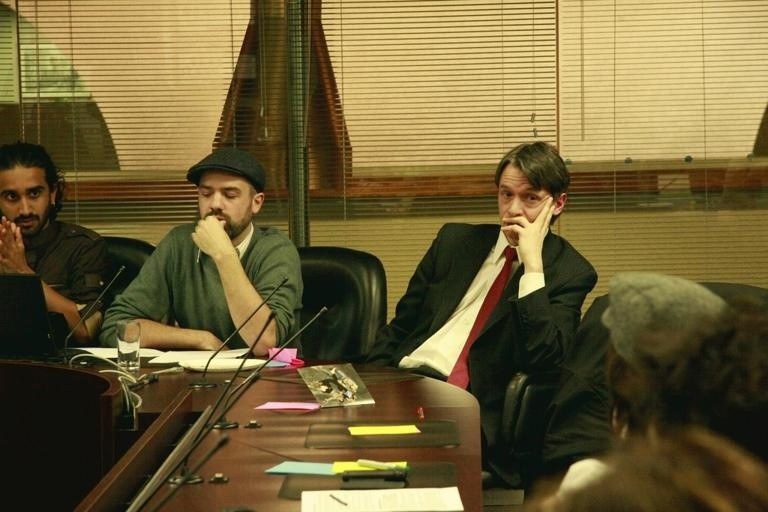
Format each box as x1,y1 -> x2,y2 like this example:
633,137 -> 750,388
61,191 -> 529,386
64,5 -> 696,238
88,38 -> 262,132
115,320 -> 141,375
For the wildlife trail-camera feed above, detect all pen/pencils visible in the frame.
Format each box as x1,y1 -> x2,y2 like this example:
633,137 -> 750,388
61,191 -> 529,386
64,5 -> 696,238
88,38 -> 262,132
128,379 -> 150,389
417,405 -> 425,424
330,494 -> 348,504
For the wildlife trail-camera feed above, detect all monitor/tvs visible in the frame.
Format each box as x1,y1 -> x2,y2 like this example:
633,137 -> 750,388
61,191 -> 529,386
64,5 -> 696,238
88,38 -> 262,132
0,274 -> 58,362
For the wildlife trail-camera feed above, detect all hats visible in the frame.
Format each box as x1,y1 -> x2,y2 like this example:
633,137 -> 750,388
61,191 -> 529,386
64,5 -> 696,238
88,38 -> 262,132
186,148 -> 265,192
602,271 -> 732,377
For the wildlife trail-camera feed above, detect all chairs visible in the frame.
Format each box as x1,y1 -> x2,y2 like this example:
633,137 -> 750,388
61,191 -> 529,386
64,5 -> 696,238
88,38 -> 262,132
295,246 -> 388,363
98,236 -> 156,316
483,369 -> 555,492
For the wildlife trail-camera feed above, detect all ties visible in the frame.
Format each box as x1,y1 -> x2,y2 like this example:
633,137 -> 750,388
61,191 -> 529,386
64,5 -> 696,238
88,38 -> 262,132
448,245 -> 518,388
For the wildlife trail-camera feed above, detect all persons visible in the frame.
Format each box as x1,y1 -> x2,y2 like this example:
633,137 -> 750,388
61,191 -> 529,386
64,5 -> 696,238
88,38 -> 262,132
364,143 -> 598,483
0,142 -> 111,349
98,147 -> 304,364
542,351 -> 767,511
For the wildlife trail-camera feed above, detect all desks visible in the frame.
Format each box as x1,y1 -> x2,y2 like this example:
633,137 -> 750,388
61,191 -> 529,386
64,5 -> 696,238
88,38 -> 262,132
0,340 -> 483,512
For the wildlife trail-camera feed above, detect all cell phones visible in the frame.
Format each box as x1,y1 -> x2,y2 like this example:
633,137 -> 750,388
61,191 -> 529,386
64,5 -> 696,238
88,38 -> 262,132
342,469 -> 407,483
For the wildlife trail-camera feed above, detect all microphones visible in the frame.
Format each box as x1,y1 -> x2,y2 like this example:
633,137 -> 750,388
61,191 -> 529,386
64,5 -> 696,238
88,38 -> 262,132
135,372 -> 260,511
150,434 -> 229,512
205,306 -> 328,429
166,310 -> 277,484
63,265 -> 127,366
188,275 -> 289,389
